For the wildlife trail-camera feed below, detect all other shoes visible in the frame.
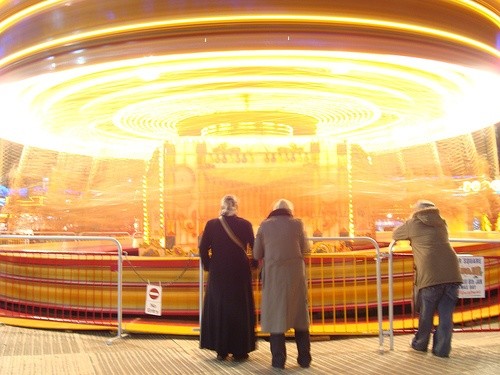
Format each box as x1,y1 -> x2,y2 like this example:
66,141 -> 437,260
233,352 -> 250,360
409,338 -> 427,352
217,354 -> 229,361
432,351 -> 449,358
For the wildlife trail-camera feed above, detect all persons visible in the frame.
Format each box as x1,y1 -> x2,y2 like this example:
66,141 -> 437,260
199,194 -> 259,361
391,199 -> 463,359
252,199 -> 312,369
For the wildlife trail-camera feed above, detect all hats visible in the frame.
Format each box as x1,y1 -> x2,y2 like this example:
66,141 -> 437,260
413,201 -> 435,210
